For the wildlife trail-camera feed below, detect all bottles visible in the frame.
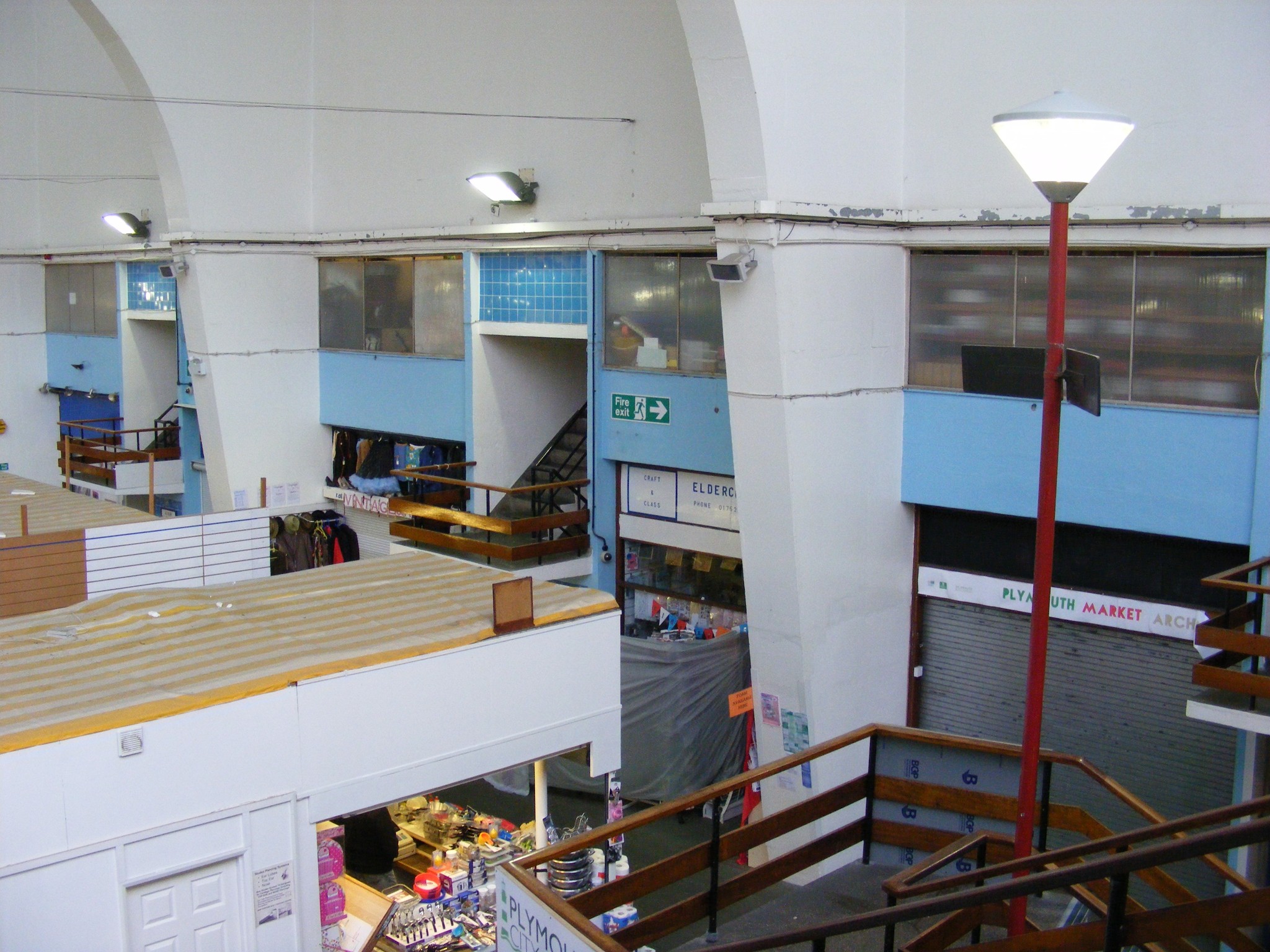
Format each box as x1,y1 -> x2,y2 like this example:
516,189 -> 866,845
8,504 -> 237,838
445,850 -> 459,874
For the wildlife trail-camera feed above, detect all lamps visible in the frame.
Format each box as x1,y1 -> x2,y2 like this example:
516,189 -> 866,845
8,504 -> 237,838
704,251 -> 756,284
84,388 -> 95,399
39,382 -> 52,393
63,385 -> 74,397
466,167 -> 538,211
99,214 -> 152,240
108,391 -> 120,403
158,260 -> 190,279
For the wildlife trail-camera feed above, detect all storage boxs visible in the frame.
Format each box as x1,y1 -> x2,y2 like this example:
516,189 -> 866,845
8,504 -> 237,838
439,857 -> 487,924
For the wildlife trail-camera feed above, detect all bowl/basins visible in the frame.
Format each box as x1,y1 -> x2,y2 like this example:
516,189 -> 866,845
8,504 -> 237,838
413,873 -> 442,899
548,848 -> 596,900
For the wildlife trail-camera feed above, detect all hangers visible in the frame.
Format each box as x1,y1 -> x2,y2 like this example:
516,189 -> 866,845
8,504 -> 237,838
270,541 -> 287,559
312,513 -> 347,540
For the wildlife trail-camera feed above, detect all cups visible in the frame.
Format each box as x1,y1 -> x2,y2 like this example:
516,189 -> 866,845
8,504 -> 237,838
433,851 -> 442,870
